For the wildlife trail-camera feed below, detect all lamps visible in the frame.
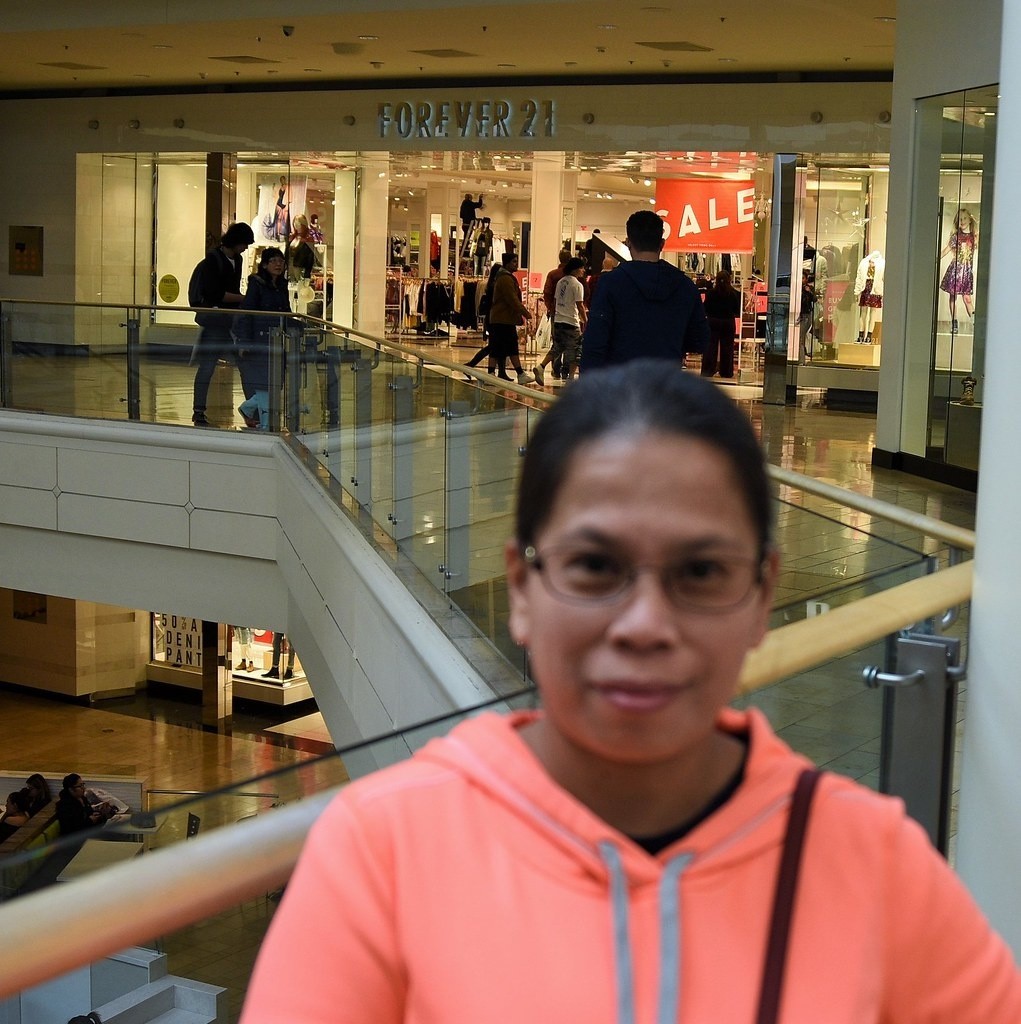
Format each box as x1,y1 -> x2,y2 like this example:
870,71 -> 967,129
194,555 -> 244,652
519,183 -> 525,187
309,198 -> 314,202
491,181 -> 498,186
320,198 -> 324,203
629,176 -> 639,183
606,193 -> 614,198
584,190 -> 589,196
596,192 -> 605,199
476,178 -> 482,185
404,205 -> 409,211
506,182 -> 513,187
409,188 -> 416,196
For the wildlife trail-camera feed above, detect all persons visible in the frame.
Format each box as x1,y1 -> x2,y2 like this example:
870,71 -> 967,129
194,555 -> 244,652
802,272 -> 818,337
696,269 -> 741,378
236,360 -> 1019,1024
801,243 -> 860,338
56,774 -> 119,855
854,251 -> 884,344
238,248 -> 293,432
274,175 -> 293,242
532,229 -> 614,386
461,253 -> 535,385
469,218 -> 494,278
937,209 -> 979,335
0,774 -> 52,848
460,193 -> 484,250
285,214 -> 323,285
192,222 -> 261,428
576,210 -> 710,375
236,626 -> 295,682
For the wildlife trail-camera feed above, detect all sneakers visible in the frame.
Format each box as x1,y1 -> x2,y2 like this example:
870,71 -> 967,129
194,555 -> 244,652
518,373 -> 534,384
562,378 -> 573,386
862,338 -> 872,344
533,364 -> 546,386
855,338 -> 864,344
961,376 -> 977,406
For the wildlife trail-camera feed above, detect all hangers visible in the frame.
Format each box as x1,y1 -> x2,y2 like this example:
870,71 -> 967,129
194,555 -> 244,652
388,276 -> 488,285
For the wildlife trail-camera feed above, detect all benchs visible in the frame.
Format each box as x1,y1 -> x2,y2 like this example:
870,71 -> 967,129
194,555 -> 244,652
0,797 -> 68,905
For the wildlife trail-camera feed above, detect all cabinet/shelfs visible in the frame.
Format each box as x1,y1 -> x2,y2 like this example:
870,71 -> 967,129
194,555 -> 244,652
738,279 -> 765,378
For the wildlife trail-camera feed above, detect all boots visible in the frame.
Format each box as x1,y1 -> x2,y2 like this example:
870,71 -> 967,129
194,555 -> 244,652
951,320 -> 959,333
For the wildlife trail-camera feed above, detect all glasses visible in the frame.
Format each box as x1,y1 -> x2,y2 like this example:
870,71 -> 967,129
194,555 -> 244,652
524,543 -> 766,615
74,782 -> 87,789
268,258 -> 285,264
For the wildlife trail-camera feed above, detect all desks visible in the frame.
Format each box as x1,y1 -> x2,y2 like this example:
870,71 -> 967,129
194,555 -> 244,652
57,841 -> 144,883
105,811 -> 169,843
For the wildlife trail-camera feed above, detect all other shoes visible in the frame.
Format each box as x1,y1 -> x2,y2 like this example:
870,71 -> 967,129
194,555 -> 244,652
462,363 -> 473,382
193,412 -> 210,426
499,372 -> 514,380
238,406 -> 257,429
551,369 -> 560,380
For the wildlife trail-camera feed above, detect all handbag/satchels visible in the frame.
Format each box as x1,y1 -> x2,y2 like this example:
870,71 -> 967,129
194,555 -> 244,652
478,285 -> 489,316
